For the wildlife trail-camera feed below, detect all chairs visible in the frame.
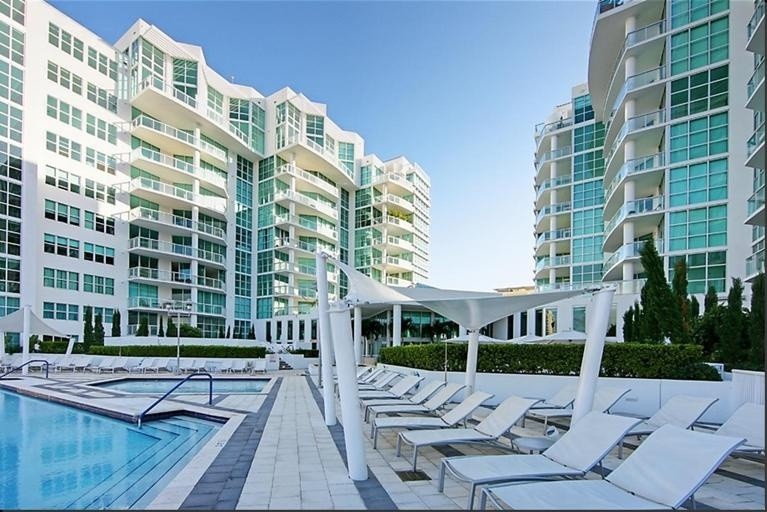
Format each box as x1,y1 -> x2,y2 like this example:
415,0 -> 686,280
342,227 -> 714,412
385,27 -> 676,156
478,421 -> 749,511
689,401 -> 765,511
321,355 -> 631,475
618,396 -> 720,460
436,410 -> 644,510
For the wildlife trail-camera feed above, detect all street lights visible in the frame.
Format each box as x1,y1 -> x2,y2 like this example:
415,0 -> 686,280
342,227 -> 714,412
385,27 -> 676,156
166,302 -> 192,374
